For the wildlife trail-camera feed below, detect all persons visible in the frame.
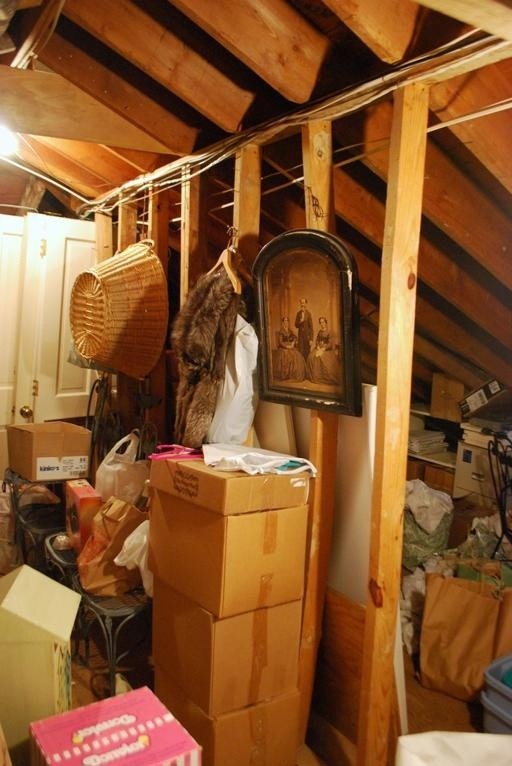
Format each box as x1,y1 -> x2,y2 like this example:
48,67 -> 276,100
295,297 -> 313,360
275,315 -> 306,382
307,317 -> 341,383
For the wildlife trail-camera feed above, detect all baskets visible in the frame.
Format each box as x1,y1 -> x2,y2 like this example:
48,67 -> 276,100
70,239 -> 169,380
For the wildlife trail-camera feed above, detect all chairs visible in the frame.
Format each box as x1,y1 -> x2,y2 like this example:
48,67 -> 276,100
4,370 -> 112,569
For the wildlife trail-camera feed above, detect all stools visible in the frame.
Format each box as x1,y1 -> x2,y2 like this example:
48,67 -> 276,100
45,531 -> 152,698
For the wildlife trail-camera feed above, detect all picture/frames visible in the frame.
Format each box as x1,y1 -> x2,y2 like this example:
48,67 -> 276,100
249,229 -> 361,419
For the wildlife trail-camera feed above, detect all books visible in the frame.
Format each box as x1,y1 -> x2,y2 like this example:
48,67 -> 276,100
460,416 -> 512,453
409,427 -> 450,456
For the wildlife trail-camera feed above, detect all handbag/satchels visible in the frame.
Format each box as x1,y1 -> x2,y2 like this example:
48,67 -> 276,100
94,432 -> 150,505
417,566 -> 512,703
77,496 -> 149,598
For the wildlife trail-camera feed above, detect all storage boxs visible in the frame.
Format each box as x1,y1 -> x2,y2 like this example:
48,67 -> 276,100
7,420 -> 90,486
29,685 -> 206,766
430,373 -> 510,422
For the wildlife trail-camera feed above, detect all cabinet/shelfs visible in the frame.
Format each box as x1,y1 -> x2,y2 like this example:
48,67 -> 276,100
408,408 -> 461,470
452,440 -> 511,513
0,209 -> 118,429
